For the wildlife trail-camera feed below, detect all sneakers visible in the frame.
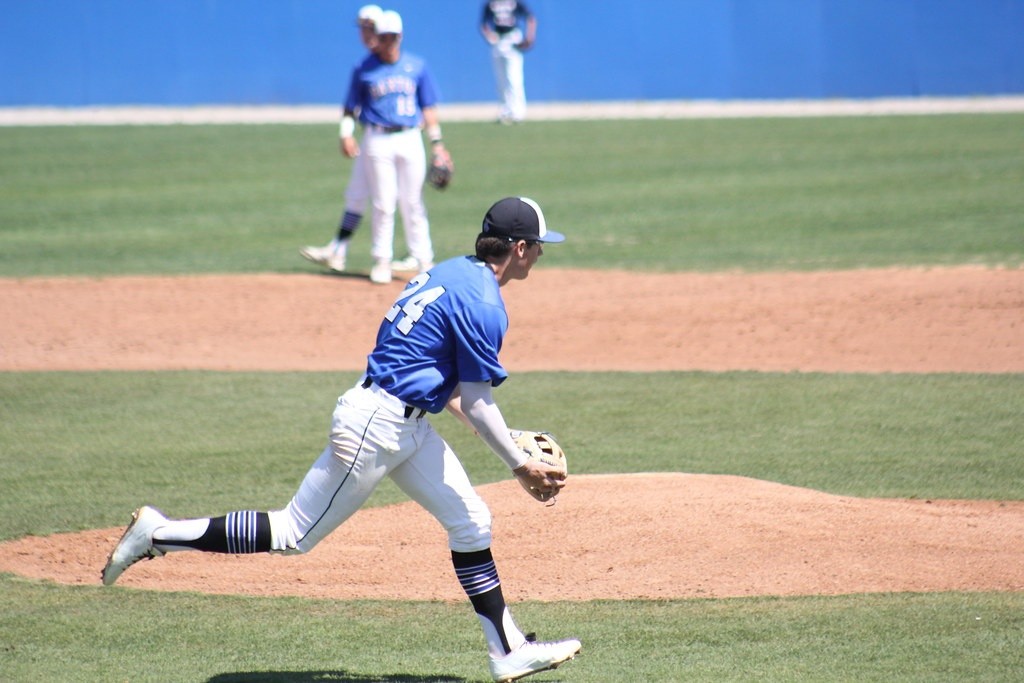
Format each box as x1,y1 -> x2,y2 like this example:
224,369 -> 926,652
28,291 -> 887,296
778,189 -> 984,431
489,638 -> 582,683
101,505 -> 171,587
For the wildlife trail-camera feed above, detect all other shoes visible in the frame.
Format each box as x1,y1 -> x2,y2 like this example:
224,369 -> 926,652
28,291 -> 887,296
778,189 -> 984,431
394,258 -> 416,270
301,243 -> 348,273
371,265 -> 393,285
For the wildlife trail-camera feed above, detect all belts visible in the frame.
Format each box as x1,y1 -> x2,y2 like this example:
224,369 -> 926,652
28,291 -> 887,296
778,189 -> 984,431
380,126 -> 402,134
363,377 -> 426,420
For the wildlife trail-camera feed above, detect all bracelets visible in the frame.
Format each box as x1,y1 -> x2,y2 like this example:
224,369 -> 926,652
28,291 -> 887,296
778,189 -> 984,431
425,125 -> 443,143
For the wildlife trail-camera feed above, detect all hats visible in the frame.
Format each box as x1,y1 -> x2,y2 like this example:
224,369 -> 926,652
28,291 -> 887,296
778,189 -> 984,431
374,10 -> 403,34
483,198 -> 565,244
358,4 -> 384,25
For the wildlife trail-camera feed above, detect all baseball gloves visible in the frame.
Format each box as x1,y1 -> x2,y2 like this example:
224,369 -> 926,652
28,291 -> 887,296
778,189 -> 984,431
511,40 -> 533,54
427,149 -> 452,191
509,429 -> 568,507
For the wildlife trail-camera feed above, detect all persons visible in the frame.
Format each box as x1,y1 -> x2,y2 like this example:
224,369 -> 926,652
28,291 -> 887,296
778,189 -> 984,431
478,0 -> 537,123
101,197 -> 583,683
298,5 -> 453,284
